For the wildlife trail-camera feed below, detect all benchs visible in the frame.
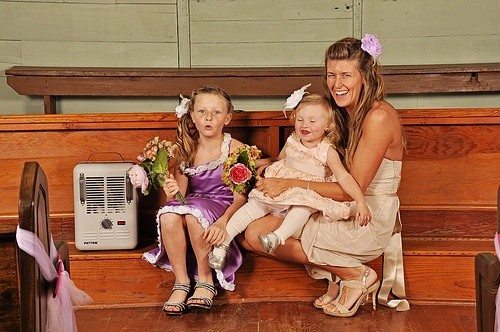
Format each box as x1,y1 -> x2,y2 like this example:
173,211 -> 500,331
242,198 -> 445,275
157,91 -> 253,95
0,108 -> 500,310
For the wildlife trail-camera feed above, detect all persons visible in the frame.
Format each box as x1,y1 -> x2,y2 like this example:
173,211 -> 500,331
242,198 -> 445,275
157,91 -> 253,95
238,31 -> 404,317
206,94 -> 371,270
159,84 -> 255,317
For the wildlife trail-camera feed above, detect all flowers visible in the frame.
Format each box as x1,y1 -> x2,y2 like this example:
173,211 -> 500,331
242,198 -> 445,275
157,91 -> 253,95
175,94 -> 191,119
126,136 -> 186,205
221,144 -> 262,195
282,83 -> 312,118
361,33 -> 382,67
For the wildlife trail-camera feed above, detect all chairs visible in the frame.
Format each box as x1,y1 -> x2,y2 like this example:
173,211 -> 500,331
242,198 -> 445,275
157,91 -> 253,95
475,184 -> 499,332
